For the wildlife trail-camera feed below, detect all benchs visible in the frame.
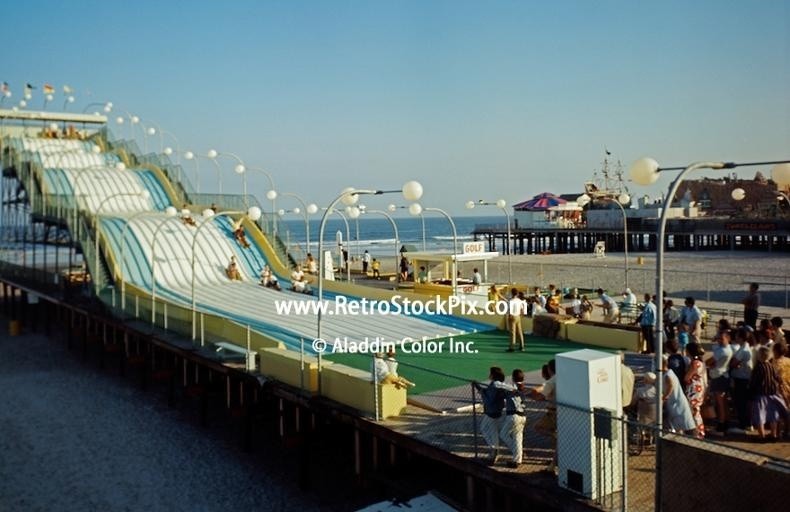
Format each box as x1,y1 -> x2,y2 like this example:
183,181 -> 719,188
320,362 -> 407,420
567,320 -> 644,353
505,312 -> 579,341
259,347 -> 334,393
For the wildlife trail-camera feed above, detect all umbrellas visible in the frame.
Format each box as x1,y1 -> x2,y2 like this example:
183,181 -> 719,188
512,192 -> 567,211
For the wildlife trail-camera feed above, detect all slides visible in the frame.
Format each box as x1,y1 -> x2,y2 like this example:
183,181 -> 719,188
0,107 -> 498,353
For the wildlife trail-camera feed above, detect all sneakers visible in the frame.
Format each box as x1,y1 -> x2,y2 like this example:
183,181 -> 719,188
487,456 -> 522,469
519,347 -> 525,352
504,348 -> 515,353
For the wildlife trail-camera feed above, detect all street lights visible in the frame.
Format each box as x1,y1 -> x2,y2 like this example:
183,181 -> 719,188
575,188 -> 630,325
629,157 -> 727,511
466,198 -> 514,299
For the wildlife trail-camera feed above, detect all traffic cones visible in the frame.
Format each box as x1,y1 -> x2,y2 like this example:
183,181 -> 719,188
637,256 -> 643,265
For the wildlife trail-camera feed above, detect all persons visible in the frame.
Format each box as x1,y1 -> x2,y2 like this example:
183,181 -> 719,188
179,204 -> 196,226
227,245 -> 790,468
234,224 -> 249,248
210,203 -> 217,213
